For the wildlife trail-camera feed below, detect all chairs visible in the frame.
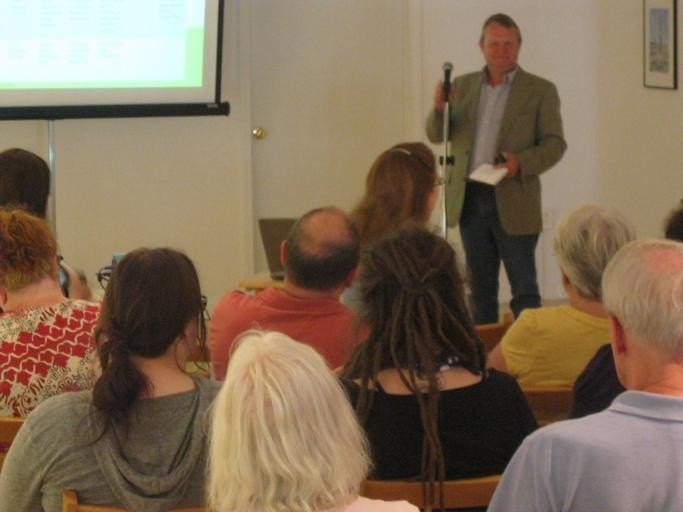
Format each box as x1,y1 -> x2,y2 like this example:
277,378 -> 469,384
473,312 -> 518,353
2,418 -> 29,456
520,387 -> 572,424
62,489 -> 205,511
362,476 -> 502,510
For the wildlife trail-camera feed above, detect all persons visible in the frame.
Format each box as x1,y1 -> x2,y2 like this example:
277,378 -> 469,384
425,14 -> 567,325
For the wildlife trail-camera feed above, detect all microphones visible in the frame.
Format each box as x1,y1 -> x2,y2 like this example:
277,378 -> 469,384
442,63 -> 452,92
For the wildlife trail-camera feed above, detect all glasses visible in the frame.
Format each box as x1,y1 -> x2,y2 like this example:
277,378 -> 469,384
433,177 -> 449,187
198,295 -> 209,310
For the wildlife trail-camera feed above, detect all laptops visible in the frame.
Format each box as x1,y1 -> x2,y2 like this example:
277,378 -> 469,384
259,218 -> 299,280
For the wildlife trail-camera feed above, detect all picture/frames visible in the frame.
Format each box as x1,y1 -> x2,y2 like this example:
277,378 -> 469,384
642,1 -> 676,91
